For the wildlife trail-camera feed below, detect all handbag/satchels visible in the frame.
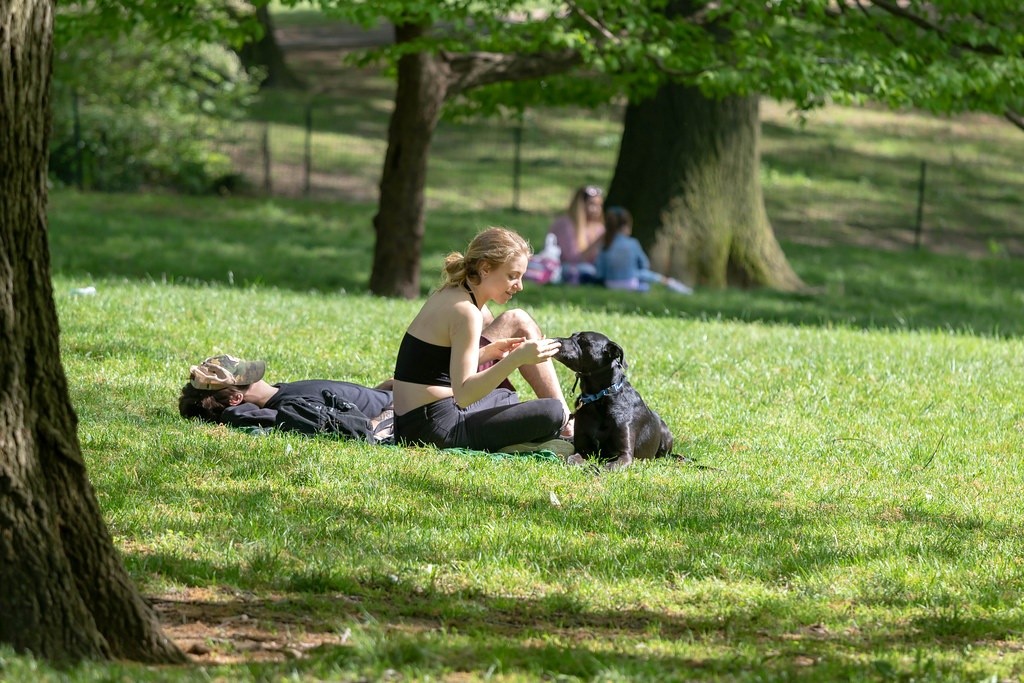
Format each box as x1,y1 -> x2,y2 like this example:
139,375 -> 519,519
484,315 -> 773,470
522,259 -> 561,284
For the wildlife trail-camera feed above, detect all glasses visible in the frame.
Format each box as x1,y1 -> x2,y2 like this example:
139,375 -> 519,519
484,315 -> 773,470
583,186 -> 602,197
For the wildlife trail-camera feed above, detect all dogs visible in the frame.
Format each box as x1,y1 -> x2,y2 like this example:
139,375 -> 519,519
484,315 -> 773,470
552,331 -> 694,468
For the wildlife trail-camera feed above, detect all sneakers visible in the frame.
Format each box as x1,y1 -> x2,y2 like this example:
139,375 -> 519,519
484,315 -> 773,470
497,439 -> 575,460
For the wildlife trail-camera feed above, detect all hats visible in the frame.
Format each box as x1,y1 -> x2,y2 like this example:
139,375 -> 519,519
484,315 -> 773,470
186,354 -> 266,391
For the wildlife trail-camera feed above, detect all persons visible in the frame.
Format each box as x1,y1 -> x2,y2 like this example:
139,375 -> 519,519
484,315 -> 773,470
549,184 -> 607,284
179,300 -> 581,445
592,205 -> 691,290
391,226 -> 577,459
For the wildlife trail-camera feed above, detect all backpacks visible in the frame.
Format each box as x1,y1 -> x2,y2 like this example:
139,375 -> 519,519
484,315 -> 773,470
276,390 -> 377,444
